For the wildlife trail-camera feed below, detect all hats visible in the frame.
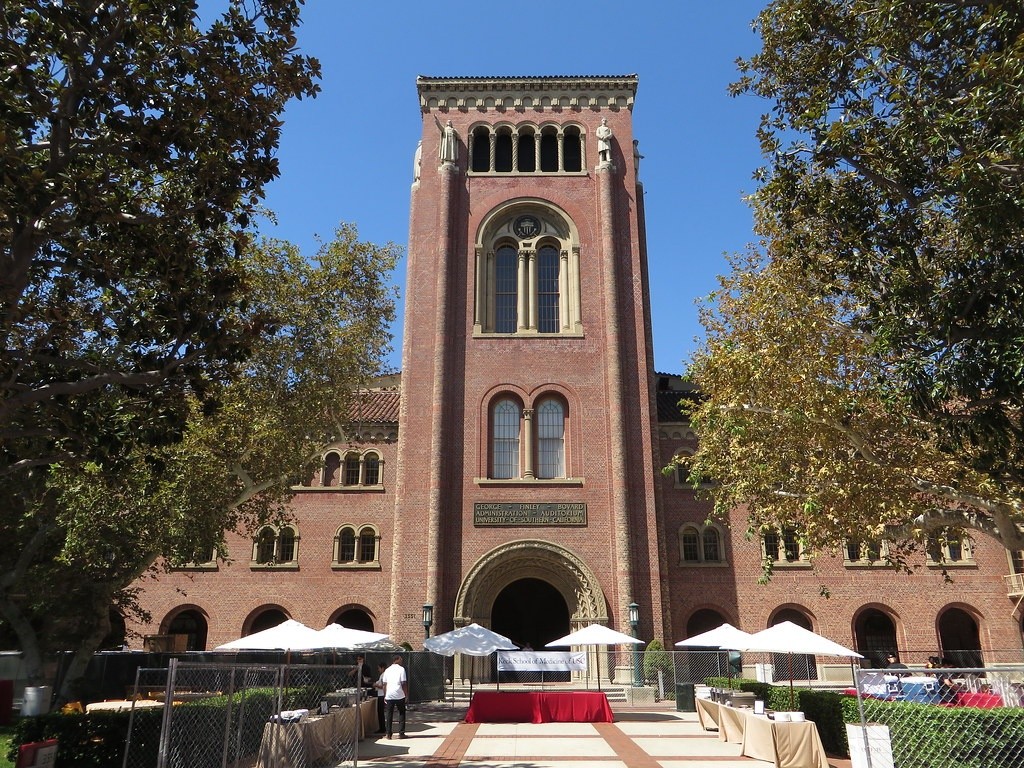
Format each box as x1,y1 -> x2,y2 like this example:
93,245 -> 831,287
886,651 -> 897,658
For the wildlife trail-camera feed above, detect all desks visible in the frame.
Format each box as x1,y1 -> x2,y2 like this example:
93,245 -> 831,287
253,696 -> 380,768
695,685 -> 830,768
465,689 -> 615,724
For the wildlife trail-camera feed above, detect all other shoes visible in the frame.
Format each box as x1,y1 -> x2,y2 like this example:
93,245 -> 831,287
388,736 -> 392,739
399,734 -> 408,739
374,729 -> 386,735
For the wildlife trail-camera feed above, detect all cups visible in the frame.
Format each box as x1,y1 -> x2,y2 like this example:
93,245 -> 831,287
790,712 -> 804,722
775,712 -> 790,721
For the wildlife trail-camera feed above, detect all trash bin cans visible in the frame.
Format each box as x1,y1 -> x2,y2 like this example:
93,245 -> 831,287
675,682 -> 697,712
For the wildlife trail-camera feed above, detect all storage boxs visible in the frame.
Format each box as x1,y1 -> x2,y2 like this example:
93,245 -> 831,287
900,676 -> 942,705
863,673 -> 899,700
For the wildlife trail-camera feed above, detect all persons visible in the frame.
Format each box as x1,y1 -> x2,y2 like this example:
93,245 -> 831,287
346,654 -> 373,696
383,655 -> 409,740
522,642 -> 533,651
372,663 -> 386,733
884,651 -> 912,677
925,656 -> 960,703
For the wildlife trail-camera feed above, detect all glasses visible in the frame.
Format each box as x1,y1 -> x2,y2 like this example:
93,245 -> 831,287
356,659 -> 361,660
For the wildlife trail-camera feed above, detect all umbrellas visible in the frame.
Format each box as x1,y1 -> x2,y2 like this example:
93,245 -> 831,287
740,621 -> 864,711
545,624 -> 646,692
215,619 -> 353,711
317,623 -> 390,665
675,623 -> 753,689
423,623 -> 520,707
